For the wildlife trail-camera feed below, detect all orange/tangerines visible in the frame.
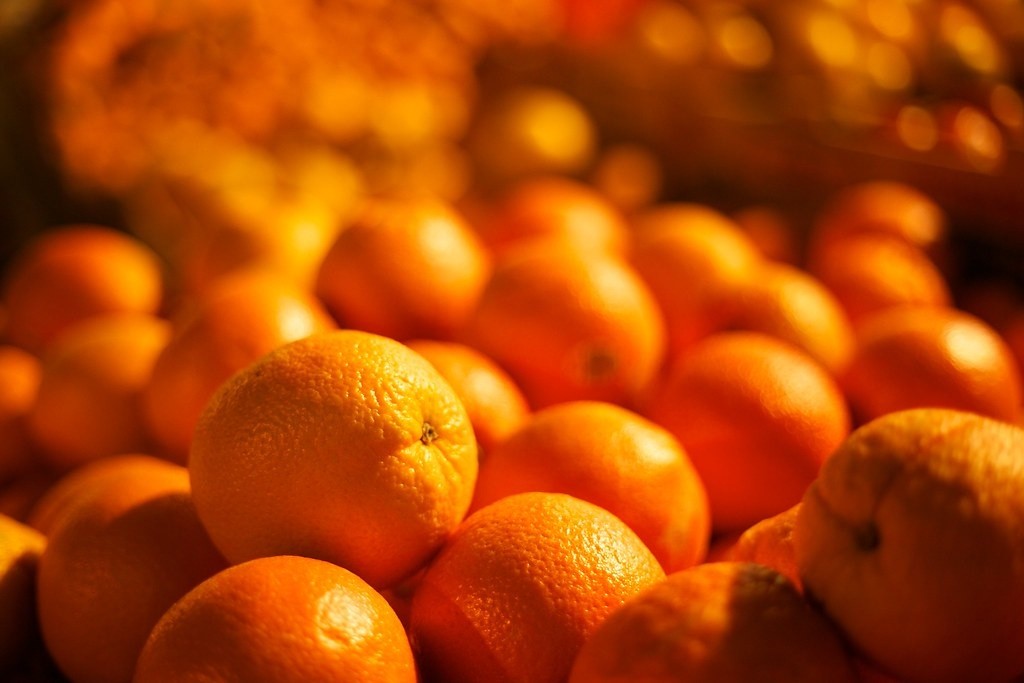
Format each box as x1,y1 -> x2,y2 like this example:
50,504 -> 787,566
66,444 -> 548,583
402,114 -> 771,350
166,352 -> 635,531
0,70 -> 1024,683
